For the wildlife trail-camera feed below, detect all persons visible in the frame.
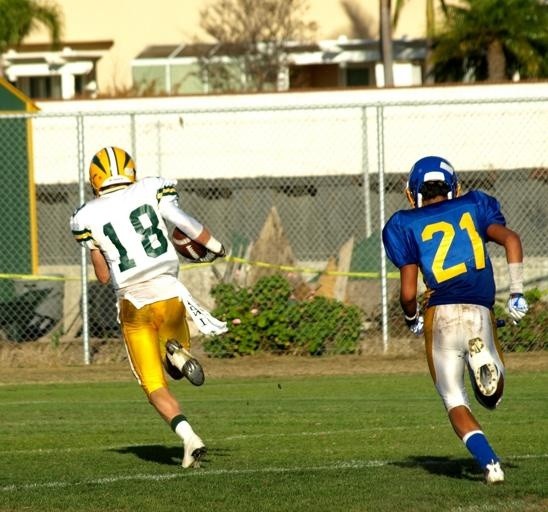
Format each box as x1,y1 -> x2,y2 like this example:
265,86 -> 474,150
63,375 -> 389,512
69,147 -> 226,470
383,156 -> 528,485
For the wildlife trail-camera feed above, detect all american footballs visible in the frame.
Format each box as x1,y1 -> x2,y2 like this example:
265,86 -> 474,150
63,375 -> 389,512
172,227 -> 218,264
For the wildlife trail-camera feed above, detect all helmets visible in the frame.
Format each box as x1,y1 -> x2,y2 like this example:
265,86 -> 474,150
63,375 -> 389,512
89,146 -> 136,194
406,156 -> 462,206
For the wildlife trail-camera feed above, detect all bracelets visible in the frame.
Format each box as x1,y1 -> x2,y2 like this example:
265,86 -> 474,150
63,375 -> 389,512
506,262 -> 525,293
204,234 -> 223,255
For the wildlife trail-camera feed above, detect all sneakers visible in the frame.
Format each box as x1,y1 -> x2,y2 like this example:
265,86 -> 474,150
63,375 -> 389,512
467,336 -> 498,398
485,462 -> 505,484
181,438 -> 207,470
164,338 -> 205,387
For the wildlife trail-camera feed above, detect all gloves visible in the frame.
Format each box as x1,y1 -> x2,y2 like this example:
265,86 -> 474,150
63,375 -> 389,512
405,307 -> 424,333
504,294 -> 528,327
